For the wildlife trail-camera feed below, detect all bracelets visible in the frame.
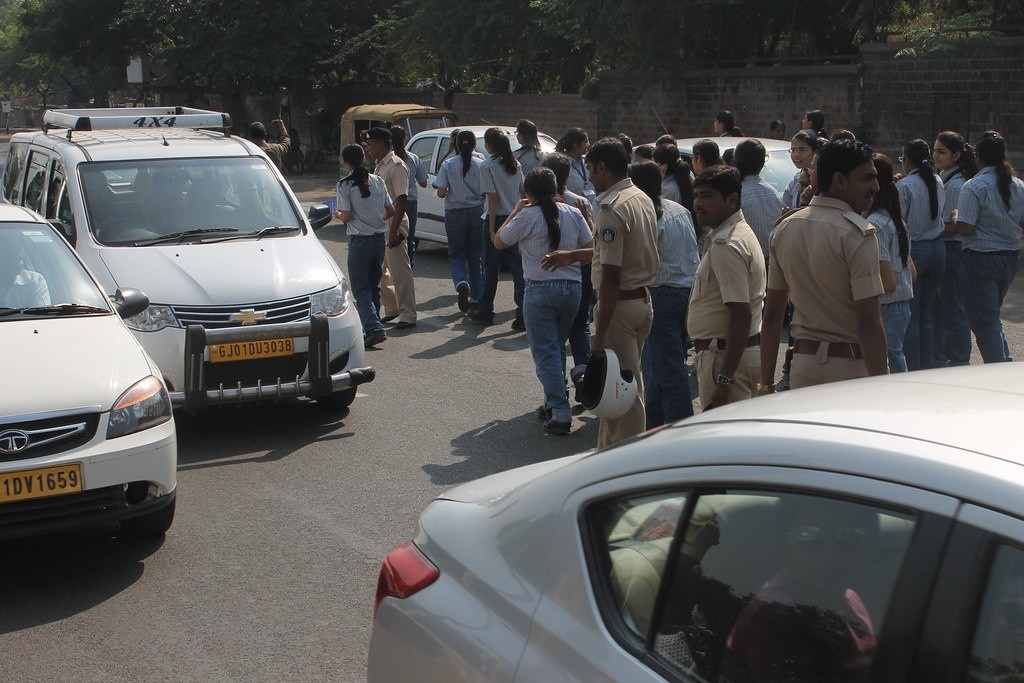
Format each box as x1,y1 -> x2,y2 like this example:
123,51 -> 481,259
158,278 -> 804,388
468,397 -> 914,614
758,382 -> 777,393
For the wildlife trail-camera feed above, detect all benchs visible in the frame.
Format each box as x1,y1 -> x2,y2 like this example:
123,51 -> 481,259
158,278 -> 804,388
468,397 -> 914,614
612,515 -> 915,683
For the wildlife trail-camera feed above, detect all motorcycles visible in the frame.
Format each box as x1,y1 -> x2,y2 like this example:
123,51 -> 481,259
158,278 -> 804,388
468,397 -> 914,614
340,104 -> 459,179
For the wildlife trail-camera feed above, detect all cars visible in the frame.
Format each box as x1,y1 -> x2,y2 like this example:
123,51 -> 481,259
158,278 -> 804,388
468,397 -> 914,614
628,136 -> 804,205
0,203 -> 179,553
363,361 -> 1024,683
405,125 -> 587,244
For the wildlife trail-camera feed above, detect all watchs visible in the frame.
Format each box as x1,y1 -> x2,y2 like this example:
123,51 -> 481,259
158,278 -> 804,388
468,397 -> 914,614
715,374 -> 733,385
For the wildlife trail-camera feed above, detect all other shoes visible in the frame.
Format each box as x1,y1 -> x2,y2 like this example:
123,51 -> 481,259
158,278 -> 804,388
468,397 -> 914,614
467,310 -> 496,326
592,285 -> 648,304
394,321 -> 416,329
456,283 -> 471,313
510,317 -> 526,331
364,329 -> 387,347
572,372 -> 585,403
535,405 -> 572,434
381,314 -> 399,323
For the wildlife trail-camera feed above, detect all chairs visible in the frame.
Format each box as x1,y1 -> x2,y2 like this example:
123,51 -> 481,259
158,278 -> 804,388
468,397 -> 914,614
87,168 -> 240,221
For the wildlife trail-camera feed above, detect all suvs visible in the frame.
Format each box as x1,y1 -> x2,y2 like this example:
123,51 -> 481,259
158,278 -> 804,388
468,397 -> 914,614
0,104 -> 369,425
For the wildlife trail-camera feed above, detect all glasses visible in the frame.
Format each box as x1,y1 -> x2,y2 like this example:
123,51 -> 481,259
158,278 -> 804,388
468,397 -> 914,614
514,130 -> 518,135
896,152 -> 908,164
788,146 -> 809,153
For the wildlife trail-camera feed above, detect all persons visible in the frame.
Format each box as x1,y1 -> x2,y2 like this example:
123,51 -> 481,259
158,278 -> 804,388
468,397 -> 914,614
335,109 -> 1024,450
719,494 -> 882,683
0,118 -> 305,315
651,598 -> 726,673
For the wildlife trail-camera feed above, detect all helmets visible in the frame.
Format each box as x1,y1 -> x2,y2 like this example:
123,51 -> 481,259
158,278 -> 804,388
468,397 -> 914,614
581,347 -> 637,419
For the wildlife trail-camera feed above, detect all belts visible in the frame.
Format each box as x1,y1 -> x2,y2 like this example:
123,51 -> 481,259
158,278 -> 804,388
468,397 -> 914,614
693,333 -> 763,355
793,339 -> 863,360
